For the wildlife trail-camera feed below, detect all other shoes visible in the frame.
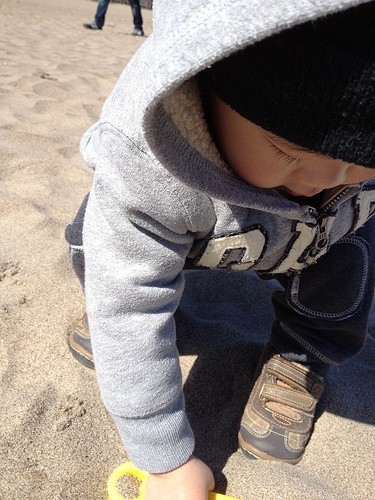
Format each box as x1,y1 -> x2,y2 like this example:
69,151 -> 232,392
132,28 -> 143,35
83,22 -> 101,30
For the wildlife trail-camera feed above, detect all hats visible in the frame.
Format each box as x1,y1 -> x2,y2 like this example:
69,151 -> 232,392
197,2 -> 375,168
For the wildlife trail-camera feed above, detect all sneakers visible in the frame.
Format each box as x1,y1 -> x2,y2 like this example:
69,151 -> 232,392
237,340 -> 323,465
68,310 -> 95,370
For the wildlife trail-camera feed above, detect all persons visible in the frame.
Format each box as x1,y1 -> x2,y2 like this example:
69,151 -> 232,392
84,0 -> 144,36
64,0 -> 375,500
